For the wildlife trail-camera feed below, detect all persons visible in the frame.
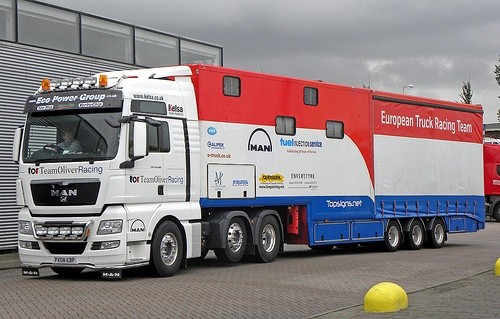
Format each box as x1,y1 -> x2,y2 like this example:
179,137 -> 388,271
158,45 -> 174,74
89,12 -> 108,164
53,126 -> 83,154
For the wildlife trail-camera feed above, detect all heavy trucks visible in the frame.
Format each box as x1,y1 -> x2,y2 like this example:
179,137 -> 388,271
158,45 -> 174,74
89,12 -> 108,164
12,63 -> 487,280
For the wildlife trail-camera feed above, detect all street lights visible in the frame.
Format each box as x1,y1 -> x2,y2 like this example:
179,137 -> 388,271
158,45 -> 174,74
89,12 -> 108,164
402,84 -> 414,94
